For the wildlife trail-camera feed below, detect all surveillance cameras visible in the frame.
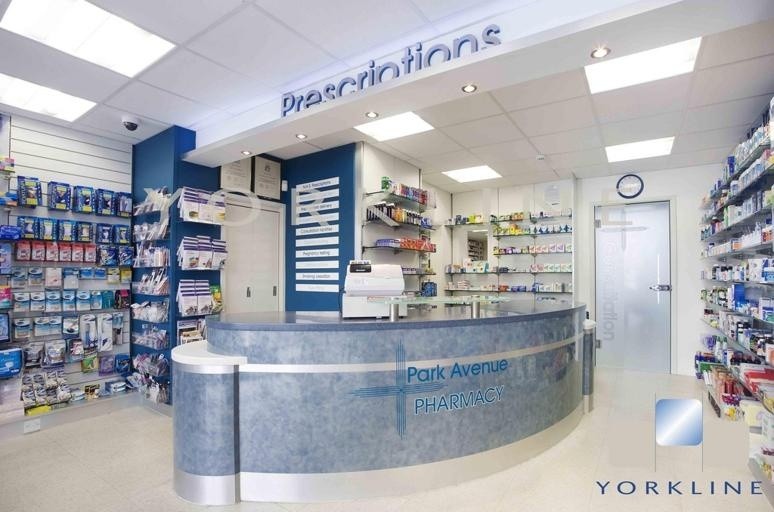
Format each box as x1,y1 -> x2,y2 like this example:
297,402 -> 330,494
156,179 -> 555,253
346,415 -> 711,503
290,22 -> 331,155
122,117 -> 142,132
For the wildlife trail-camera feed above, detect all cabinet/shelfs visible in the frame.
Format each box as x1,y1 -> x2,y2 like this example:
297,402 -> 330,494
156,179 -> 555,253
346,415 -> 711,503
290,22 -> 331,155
445,214 -> 574,294
362,190 -> 437,275
694,133 -> 774,415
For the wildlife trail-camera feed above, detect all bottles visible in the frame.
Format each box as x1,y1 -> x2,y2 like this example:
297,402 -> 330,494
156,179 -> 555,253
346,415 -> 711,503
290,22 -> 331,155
389,296 -> 399,321
366,201 -> 421,226
768,96 -> 774,155
378,176 -> 399,196
470,295 -> 479,316
694,149 -> 774,420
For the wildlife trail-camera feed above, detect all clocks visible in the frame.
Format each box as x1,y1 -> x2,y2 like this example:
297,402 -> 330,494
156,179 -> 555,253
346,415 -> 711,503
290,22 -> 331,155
616,174 -> 644,199
